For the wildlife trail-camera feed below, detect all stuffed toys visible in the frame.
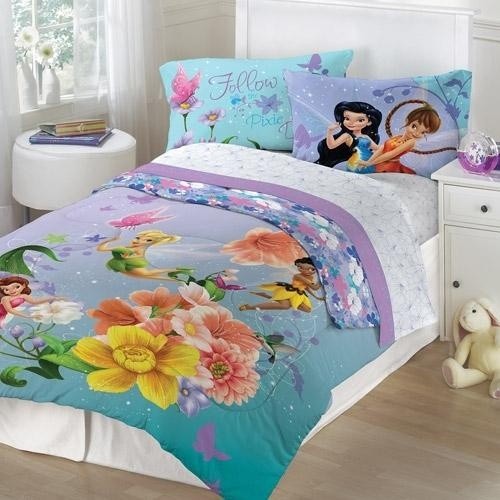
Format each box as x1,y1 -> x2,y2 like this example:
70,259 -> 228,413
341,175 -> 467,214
441,296 -> 500,399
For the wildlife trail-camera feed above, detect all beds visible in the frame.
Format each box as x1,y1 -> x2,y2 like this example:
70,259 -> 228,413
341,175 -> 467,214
0,0 -> 472,500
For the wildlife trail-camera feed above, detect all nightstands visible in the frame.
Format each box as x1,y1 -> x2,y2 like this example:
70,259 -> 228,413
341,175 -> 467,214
431,160 -> 500,342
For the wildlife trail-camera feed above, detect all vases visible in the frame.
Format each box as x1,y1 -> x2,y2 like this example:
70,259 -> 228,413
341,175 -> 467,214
16,69 -> 38,111
42,69 -> 60,104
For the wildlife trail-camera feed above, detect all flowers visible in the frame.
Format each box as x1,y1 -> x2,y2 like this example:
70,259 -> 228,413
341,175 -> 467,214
14,24 -> 71,69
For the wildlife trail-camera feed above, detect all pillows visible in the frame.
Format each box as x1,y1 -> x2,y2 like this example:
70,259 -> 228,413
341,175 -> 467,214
283,68 -> 472,179
160,50 -> 353,153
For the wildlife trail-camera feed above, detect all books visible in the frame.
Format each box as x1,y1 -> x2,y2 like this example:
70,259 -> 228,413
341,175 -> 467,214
39,118 -> 106,138
29,128 -> 112,146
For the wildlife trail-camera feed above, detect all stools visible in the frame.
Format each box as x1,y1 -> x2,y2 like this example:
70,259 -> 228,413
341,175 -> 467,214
12,129 -> 136,225
